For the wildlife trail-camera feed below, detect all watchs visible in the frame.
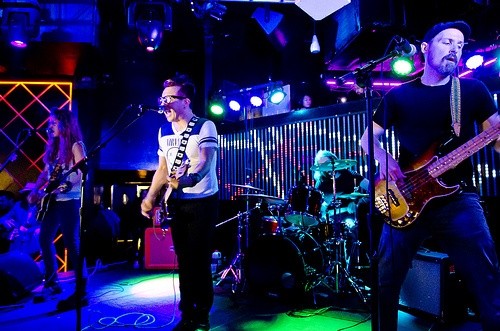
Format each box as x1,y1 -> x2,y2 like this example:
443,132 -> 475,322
189,173 -> 200,186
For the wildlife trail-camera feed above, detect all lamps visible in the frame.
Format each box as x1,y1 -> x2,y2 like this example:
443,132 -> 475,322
0,1 -> 43,49
249,84 -> 287,107
208,91 -> 245,116
390,54 -> 417,79
461,42 -> 484,72
125,1 -> 172,53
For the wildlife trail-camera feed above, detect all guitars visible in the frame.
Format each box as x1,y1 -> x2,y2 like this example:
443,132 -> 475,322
368,121 -> 500,231
36,180 -> 73,221
150,163 -> 191,228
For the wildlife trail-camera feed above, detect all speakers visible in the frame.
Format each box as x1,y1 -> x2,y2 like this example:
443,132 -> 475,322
398,247 -> 469,318
144,228 -> 179,270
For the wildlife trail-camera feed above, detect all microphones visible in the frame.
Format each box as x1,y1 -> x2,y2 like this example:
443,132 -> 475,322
24,128 -> 52,134
395,35 -> 418,57
130,103 -> 165,114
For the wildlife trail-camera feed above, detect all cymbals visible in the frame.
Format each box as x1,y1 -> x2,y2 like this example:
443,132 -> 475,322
310,158 -> 358,172
231,183 -> 262,192
240,194 -> 282,200
338,192 -> 370,198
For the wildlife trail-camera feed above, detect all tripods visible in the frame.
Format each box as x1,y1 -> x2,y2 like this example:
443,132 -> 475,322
216,200 -> 264,294
306,157 -> 373,306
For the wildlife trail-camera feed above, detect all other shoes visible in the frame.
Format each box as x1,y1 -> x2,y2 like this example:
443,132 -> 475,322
57,275 -> 89,308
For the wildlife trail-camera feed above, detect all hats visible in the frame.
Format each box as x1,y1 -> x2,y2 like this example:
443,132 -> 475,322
423,20 -> 471,44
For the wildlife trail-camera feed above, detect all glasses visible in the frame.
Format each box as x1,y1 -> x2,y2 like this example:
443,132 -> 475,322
158,95 -> 186,104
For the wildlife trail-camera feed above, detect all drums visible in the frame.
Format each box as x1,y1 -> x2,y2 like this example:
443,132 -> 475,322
319,221 -> 346,243
242,229 -> 326,306
261,201 -> 287,223
284,184 -> 324,228
265,221 -> 287,236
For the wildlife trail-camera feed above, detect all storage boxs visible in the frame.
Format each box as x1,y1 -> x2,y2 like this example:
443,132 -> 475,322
144,228 -> 178,270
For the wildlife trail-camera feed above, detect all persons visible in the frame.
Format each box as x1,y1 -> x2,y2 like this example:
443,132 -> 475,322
0,183 -> 45,274
141,79 -> 219,331
28,107 -> 88,311
361,21 -> 500,331
298,94 -> 312,109
314,151 -> 369,220
